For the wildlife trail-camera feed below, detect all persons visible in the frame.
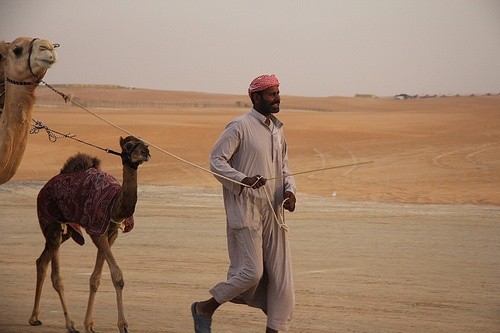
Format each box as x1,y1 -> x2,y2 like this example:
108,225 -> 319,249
191,73 -> 297,333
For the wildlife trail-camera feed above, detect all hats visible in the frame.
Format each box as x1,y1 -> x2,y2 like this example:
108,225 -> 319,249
249,74 -> 280,94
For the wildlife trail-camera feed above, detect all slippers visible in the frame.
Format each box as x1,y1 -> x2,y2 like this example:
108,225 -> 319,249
191,302 -> 212,333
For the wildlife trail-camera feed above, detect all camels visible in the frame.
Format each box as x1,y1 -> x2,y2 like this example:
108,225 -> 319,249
29,136 -> 151,333
0,37 -> 57,186
